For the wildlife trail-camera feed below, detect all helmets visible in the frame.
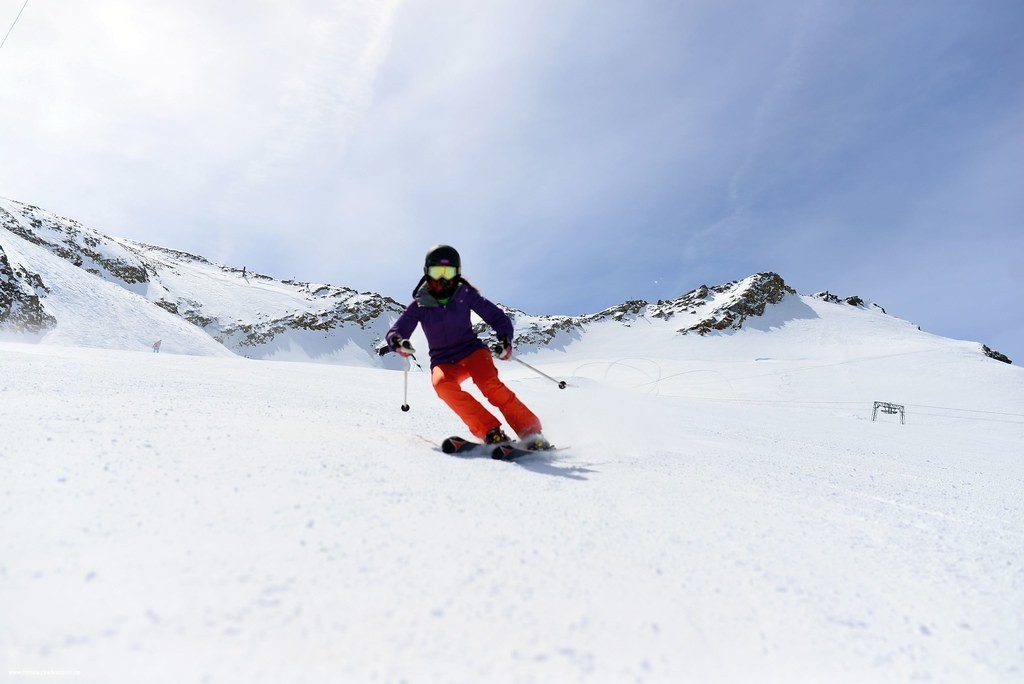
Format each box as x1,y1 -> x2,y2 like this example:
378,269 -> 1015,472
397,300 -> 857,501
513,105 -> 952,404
423,244 -> 462,293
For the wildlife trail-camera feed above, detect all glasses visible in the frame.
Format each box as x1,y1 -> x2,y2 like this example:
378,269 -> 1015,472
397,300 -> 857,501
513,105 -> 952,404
427,267 -> 457,280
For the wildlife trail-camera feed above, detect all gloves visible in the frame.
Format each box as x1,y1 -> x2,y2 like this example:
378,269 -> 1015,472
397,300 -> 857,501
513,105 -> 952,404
395,339 -> 416,357
494,339 -> 512,360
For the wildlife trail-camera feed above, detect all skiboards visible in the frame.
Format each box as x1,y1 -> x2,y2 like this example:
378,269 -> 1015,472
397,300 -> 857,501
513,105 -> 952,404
441,436 -> 556,462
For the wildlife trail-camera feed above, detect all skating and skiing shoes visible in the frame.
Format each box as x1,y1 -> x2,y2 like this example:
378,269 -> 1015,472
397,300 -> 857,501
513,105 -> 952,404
484,429 -> 517,452
526,431 -> 549,453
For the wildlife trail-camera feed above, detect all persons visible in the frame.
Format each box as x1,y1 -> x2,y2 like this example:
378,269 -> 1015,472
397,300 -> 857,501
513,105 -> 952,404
387,244 -> 552,451
152,339 -> 163,353
